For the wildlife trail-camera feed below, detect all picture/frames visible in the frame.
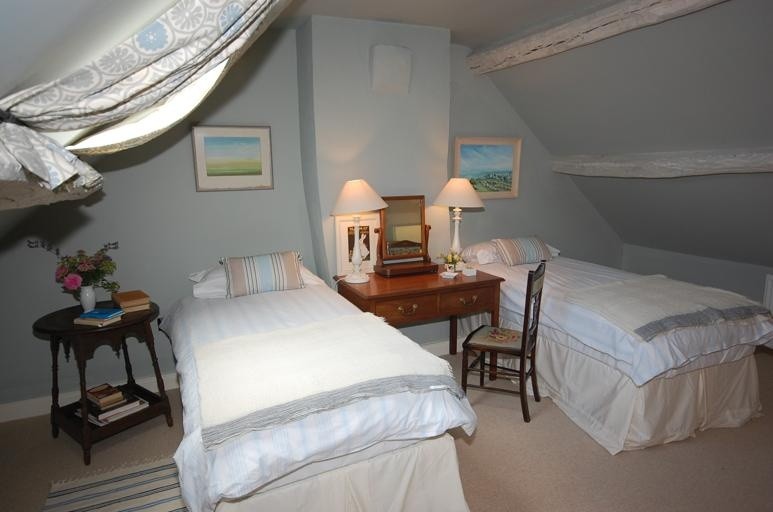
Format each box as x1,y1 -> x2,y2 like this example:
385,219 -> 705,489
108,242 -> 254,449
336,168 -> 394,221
334,220 -> 379,276
190,122 -> 276,192
452,134 -> 521,201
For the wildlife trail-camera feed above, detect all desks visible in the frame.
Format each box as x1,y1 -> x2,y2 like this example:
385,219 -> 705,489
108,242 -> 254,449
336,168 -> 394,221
32,299 -> 174,467
334,269 -> 506,382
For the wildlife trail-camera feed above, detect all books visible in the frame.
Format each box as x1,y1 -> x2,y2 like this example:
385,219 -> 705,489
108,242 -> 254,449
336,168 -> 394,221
80,306 -> 125,319
73,318 -> 122,329
122,304 -> 151,313
113,289 -> 152,309
73,381 -> 150,428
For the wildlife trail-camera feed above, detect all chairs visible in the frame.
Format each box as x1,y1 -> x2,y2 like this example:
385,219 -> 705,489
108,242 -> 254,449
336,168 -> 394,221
460,259 -> 558,424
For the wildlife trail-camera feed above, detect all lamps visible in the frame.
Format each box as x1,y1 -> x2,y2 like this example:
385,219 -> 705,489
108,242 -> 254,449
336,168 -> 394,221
335,179 -> 390,282
434,178 -> 485,267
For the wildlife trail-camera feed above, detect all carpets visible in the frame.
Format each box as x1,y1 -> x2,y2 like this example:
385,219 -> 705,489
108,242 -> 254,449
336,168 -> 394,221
43,455 -> 190,512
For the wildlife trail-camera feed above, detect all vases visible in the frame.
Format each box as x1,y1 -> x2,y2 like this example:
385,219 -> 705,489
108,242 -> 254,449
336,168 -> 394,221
78,285 -> 98,315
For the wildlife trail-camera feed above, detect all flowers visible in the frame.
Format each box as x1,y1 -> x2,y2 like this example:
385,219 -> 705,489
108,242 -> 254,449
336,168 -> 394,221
28,233 -> 125,292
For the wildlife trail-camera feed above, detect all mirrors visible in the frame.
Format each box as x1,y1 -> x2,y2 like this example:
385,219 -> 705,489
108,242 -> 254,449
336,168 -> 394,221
376,193 -> 440,277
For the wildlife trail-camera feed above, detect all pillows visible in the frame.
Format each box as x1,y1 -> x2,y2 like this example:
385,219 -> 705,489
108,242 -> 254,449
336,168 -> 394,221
462,235 -> 558,272
188,248 -> 325,301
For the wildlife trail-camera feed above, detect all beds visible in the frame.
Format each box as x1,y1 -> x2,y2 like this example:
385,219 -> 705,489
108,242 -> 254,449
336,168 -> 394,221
460,253 -> 763,445
162,292 -> 469,512
